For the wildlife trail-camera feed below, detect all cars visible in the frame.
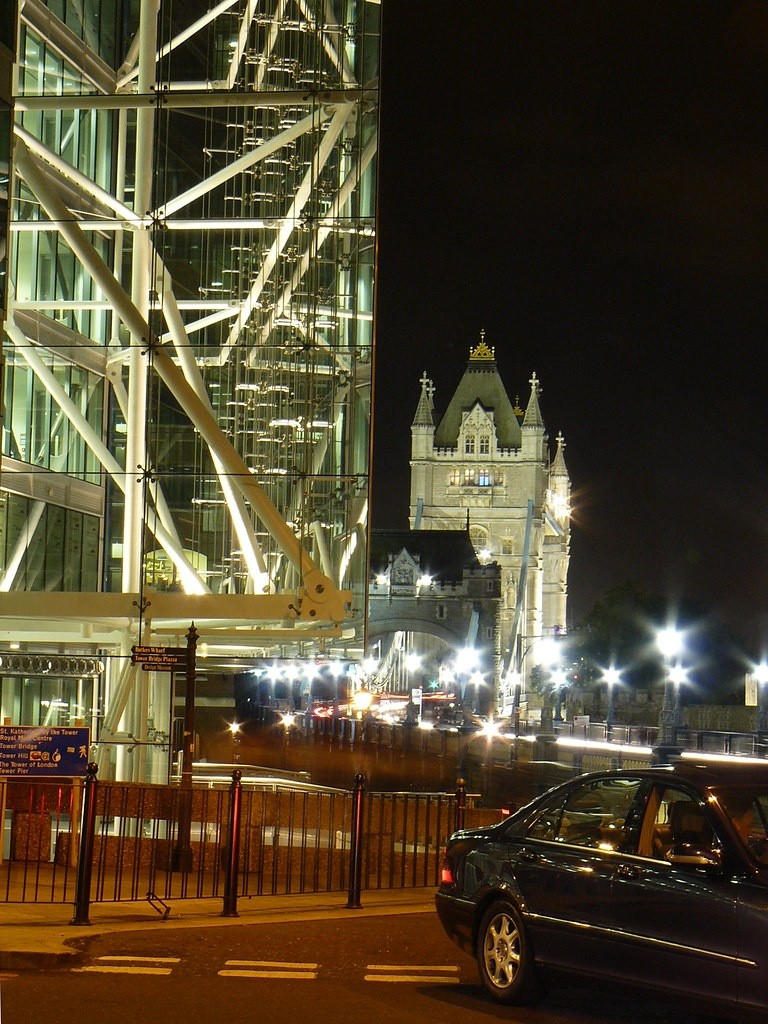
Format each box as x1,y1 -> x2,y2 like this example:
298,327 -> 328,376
433,759 -> 768,1017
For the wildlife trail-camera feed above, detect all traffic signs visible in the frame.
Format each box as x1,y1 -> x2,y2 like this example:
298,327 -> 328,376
130,645 -> 188,673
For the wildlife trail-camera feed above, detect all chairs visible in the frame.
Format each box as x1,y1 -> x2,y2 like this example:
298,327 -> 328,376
669,801 -> 714,849
